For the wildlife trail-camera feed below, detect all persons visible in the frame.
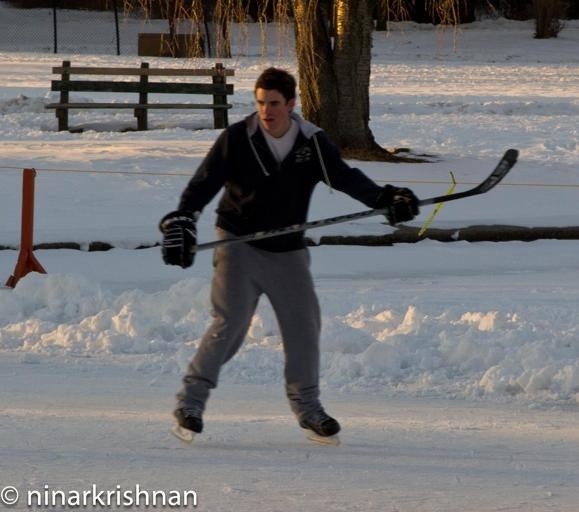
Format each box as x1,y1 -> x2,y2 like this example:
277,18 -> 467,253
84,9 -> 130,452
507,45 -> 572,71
158,66 -> 421,438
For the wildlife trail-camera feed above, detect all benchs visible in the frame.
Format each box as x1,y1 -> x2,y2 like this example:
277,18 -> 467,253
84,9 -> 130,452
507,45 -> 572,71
44,60 -> 234,130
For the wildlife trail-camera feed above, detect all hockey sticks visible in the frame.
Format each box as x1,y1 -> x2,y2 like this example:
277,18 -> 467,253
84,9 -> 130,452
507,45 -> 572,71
190,149 -> 518,254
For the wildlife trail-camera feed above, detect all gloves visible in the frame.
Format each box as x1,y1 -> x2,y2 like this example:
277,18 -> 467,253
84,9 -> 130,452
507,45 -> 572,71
374,185 -> 418,226
159,210 -> 197,268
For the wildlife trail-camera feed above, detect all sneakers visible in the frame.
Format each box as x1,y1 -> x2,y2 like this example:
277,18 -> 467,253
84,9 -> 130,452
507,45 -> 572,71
174,408 -> 203,433
299,408 -> 341,436
